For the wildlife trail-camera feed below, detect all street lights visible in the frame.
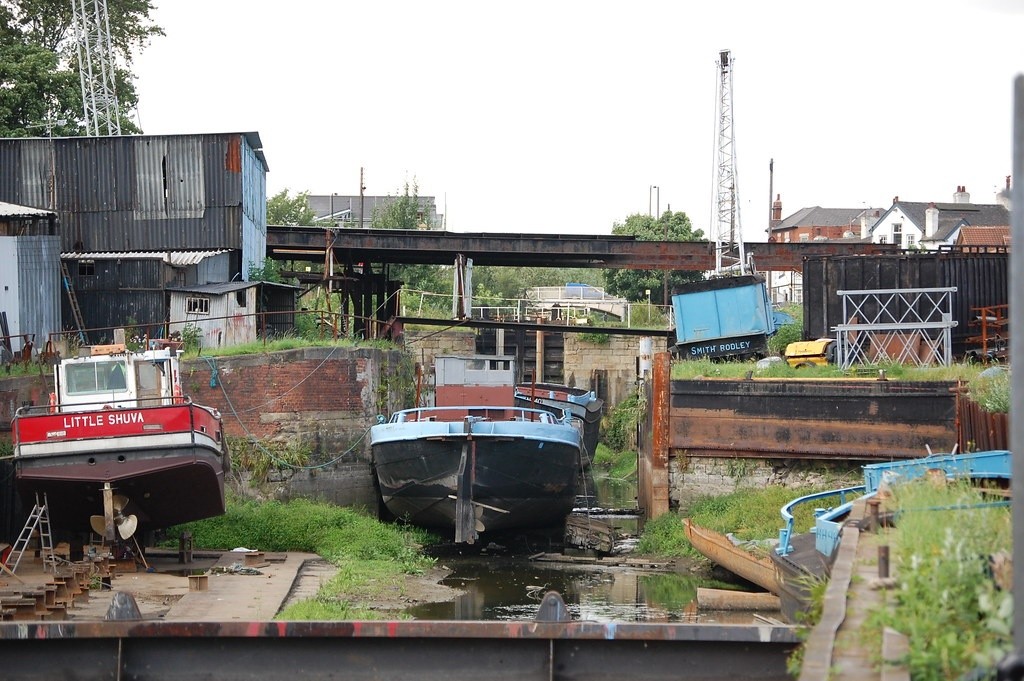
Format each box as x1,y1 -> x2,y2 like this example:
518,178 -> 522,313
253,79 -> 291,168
329,192 -> 338,221
652,185 -> 660,219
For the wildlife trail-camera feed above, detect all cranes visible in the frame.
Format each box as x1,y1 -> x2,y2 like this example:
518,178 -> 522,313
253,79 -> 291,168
702,47 -> 758,279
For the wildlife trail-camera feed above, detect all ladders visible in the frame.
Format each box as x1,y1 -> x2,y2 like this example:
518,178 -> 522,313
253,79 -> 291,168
0,490 -> 59,576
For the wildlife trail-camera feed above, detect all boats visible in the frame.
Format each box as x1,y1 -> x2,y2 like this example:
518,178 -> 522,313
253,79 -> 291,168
770,448 -> 1013,629
365,353 -> 603,550
12,341 -> 229,553
